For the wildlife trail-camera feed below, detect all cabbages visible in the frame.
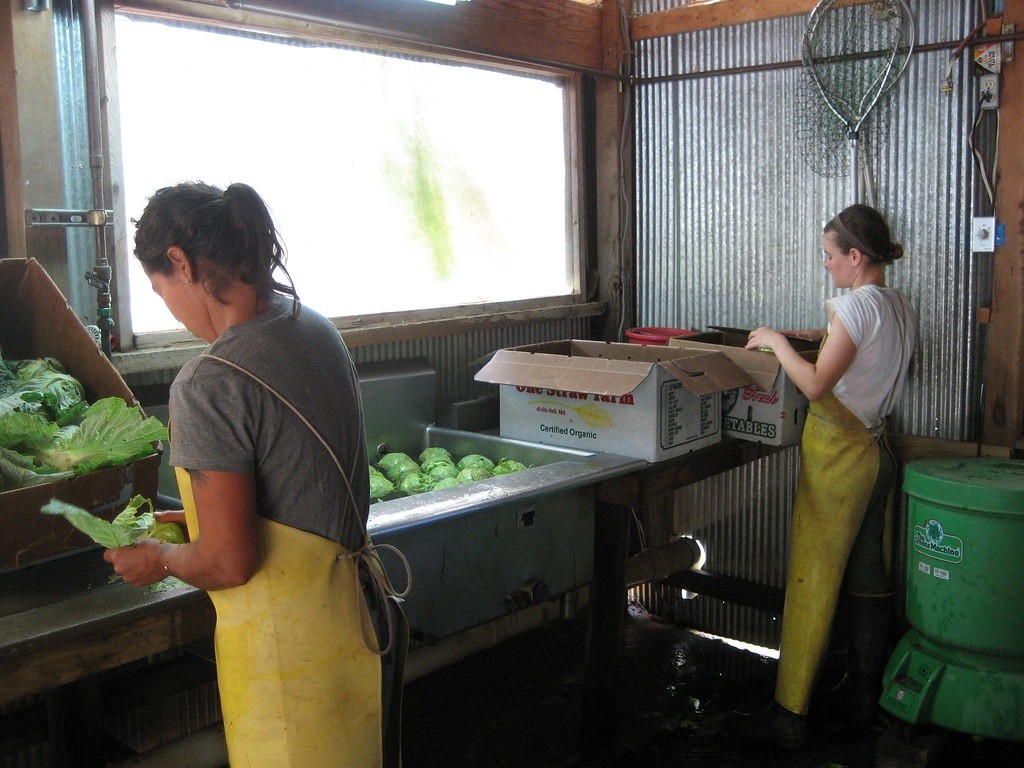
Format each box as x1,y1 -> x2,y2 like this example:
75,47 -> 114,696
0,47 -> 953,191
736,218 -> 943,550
368,447 -> 529,495
0,352 -> 186,592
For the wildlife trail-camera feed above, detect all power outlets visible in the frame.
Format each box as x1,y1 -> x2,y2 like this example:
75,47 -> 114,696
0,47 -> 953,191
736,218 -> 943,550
980,74 -> 999,110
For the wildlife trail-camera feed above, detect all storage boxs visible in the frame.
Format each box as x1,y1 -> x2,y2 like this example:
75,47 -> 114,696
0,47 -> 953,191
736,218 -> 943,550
0,257 -> 164,574
0,728 -> 49,768
474,325 -> 827,463
102,647 -> 223,754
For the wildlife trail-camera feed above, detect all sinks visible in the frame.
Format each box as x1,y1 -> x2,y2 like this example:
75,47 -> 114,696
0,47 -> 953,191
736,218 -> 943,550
0,492 -> 215,709
367,428 -> 652,692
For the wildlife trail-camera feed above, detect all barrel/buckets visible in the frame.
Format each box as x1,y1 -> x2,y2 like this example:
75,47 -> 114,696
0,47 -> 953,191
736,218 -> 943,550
625,327 -> 697,347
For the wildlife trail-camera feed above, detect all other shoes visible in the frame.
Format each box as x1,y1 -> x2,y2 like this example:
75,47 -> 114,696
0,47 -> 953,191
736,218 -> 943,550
720,702 -> 808,748
827,649 -> 873,694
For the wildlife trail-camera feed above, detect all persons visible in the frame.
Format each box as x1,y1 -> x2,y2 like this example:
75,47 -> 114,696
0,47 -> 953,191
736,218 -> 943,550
747,202 -> 914,725
101,183 -> 413,768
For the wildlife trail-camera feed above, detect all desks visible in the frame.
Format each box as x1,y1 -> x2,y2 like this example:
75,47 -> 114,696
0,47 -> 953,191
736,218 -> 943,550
586,435 -> 798,675
0,597 -> 216,768
